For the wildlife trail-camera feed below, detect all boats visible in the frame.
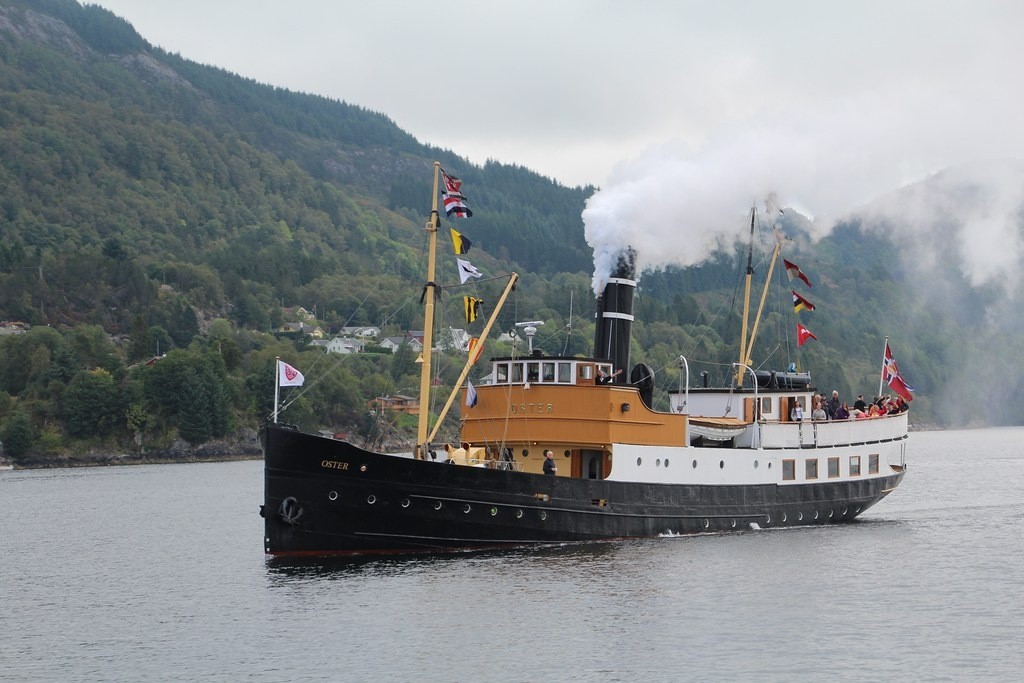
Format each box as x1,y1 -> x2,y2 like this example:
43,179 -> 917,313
258,158 -> 908,558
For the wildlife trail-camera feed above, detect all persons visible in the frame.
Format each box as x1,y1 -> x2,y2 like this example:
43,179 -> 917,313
595,369 -> 623,385
791,400 -> 804,422
812,391 -> 909,420
543,451 -> 555,475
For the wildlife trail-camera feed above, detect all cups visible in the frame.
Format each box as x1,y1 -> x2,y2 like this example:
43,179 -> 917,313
554,468 -> 557,472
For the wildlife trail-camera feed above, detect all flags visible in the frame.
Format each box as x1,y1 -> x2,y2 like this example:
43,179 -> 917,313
783,258 -> 811,288
468,337 -> 483,363
451,229 -> 472,254
466,381 -> 478,408
441,168 -> 467,202
797,324 -> 817,346
792,290 -> 816,314
884,343 -> 915,403
441,190 -> 473,218
457,258 -> 482,284
279,360 -> 304,386
464,296 -> 483,324
